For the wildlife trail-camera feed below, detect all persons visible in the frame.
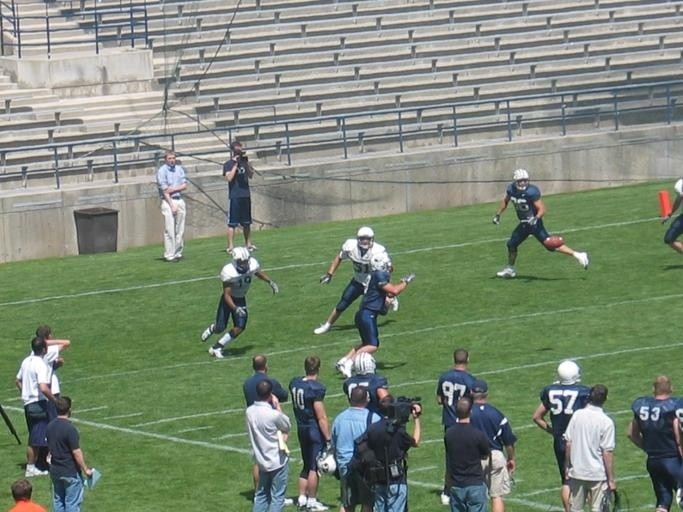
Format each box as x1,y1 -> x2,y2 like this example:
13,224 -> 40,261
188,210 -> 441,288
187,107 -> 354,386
221,139 -> 258,255
44,394 -> 93,512
628,375 -> 683,512
436,348 -> 517,512
333,251 -> 416,377
533,361 -> 616,512
490,167 -> 589,281
155,151 -> 189,263
28,324 -> 71,469
200,245 -> 279,359
659,176 -> 683,253
311,225 -> 393,334
242,354 -> 422,512
4,478 -> 48,512
13,337 -> 60,477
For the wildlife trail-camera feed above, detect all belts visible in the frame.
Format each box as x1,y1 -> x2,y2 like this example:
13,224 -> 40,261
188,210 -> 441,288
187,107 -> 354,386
162,196 -> 180,200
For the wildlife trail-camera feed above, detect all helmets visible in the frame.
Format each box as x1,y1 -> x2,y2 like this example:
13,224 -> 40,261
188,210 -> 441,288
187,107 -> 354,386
555,361 -> 581,387
357,226 -> 374,249
231,246 -> 250,273
513,169 -> 529,191
371,254 -> 392,272
316,451 -> 338,474
354,352 -> 376,375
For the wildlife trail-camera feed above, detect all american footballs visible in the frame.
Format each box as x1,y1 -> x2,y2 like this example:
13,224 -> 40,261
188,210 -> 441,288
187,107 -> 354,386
544,235 -> 563,248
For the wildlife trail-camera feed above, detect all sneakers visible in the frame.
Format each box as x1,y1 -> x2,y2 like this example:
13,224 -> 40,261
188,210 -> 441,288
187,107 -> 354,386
306,500 -> 328,512
201,326 -> 212,342
208,346 -> 223,358
313,324 -> 328,334
340,363 -> 351,377
24,468 -> 48,478
296,498 -> 304,511
496,268 -> 516,278
577,253 -> 588,268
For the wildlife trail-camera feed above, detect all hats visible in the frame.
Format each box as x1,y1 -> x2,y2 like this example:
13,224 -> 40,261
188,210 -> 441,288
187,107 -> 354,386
471,380 -> 488,393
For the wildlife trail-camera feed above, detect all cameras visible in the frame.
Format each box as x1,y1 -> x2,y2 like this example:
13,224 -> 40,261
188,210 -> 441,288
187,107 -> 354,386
236,151 -> 248,163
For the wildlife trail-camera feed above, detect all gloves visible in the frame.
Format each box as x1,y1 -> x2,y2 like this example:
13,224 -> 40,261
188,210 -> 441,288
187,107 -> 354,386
235,306 -> 246,316
319,275 -> 331,287
527,216 -> 538,226
493,214 -> 500,224
271,283 -> 278,295
401,274 -> 415,285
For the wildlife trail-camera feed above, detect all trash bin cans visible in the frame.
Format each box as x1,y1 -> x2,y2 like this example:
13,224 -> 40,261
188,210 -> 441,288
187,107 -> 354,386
72,207 -> 117,255
